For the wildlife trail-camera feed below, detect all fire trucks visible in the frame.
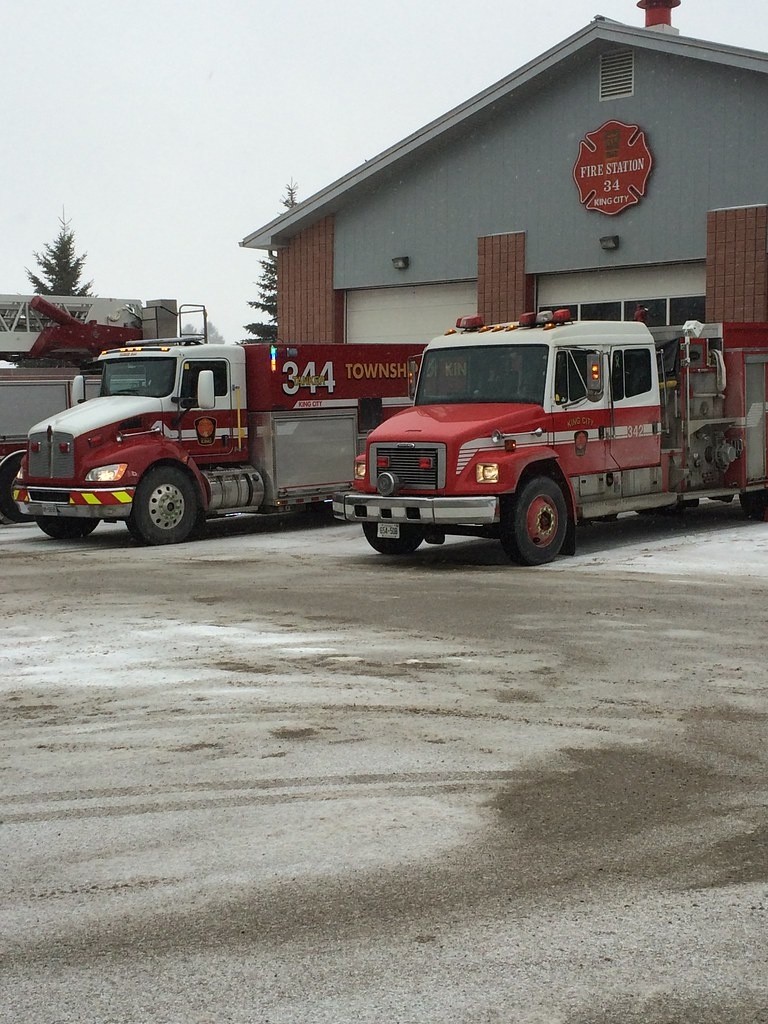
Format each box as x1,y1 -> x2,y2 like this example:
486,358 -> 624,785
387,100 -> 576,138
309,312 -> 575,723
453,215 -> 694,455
8,337 -> 517,547
329,307 -> 768,568
0,293 -> 208,523
